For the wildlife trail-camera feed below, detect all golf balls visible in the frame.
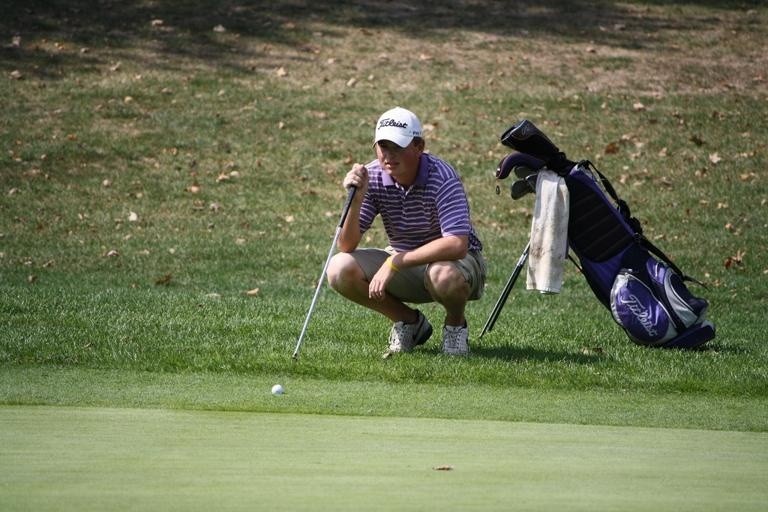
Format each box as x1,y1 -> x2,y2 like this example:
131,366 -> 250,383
271,384 -> 285,397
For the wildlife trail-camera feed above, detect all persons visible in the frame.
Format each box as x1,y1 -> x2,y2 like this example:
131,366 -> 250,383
326,106 -> 488,354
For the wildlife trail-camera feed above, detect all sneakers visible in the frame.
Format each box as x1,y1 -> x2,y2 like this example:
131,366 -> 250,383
382,309 -> 432,359
441,316 -> 471,356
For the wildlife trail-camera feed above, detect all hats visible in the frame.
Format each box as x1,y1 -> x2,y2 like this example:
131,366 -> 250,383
372,106 -> 423,149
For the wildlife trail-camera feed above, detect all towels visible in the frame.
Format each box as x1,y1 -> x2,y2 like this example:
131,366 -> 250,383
527,171 -> 571,294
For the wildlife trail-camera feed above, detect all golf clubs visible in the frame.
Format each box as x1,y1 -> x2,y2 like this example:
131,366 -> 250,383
495,151 -> 552,200
292,185 -> 356,361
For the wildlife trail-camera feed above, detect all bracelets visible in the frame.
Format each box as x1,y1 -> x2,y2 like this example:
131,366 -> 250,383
386,255 -> 400,273
